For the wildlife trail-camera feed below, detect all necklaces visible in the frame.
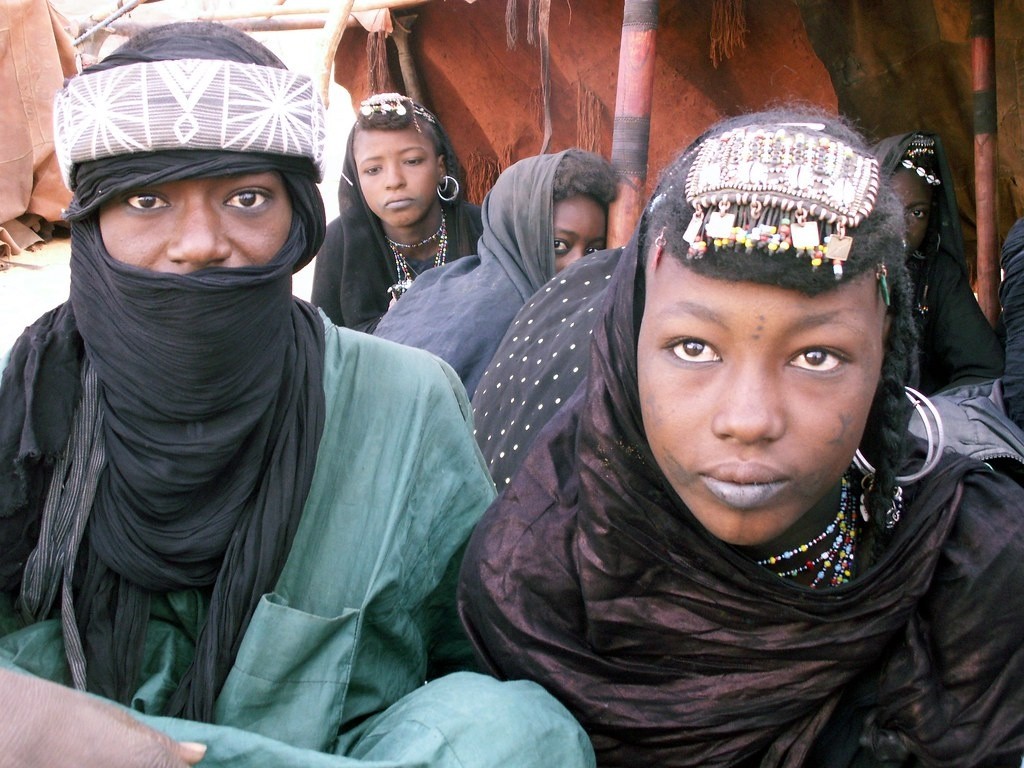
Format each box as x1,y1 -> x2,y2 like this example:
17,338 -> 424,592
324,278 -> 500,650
386,210 -> 448,286
756,473 -> 858,587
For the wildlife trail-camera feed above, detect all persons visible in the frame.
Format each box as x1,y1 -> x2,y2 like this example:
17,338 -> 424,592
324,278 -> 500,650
1,1 -> 1024,768
